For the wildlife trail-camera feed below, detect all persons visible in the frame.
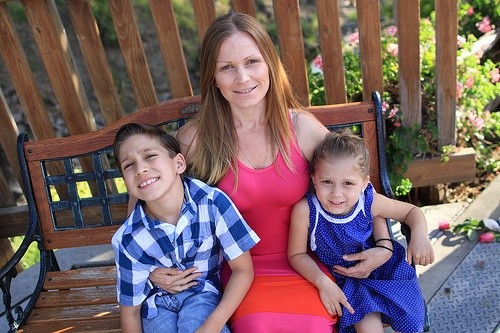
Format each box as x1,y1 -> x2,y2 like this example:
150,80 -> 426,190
149,12 -> 395,333
111,122 -> 261,333
288,128 -> 434,333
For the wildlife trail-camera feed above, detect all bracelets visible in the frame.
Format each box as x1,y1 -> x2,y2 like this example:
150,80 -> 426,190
375,239 -> 394,253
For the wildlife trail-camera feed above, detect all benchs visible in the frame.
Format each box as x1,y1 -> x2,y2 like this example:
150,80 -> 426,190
0,90 -> 430,333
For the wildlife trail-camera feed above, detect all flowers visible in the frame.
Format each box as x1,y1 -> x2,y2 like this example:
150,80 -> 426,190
439,216 -> 500,243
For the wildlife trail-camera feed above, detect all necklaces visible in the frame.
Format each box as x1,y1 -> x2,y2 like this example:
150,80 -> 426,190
238,138 -> 269,169
